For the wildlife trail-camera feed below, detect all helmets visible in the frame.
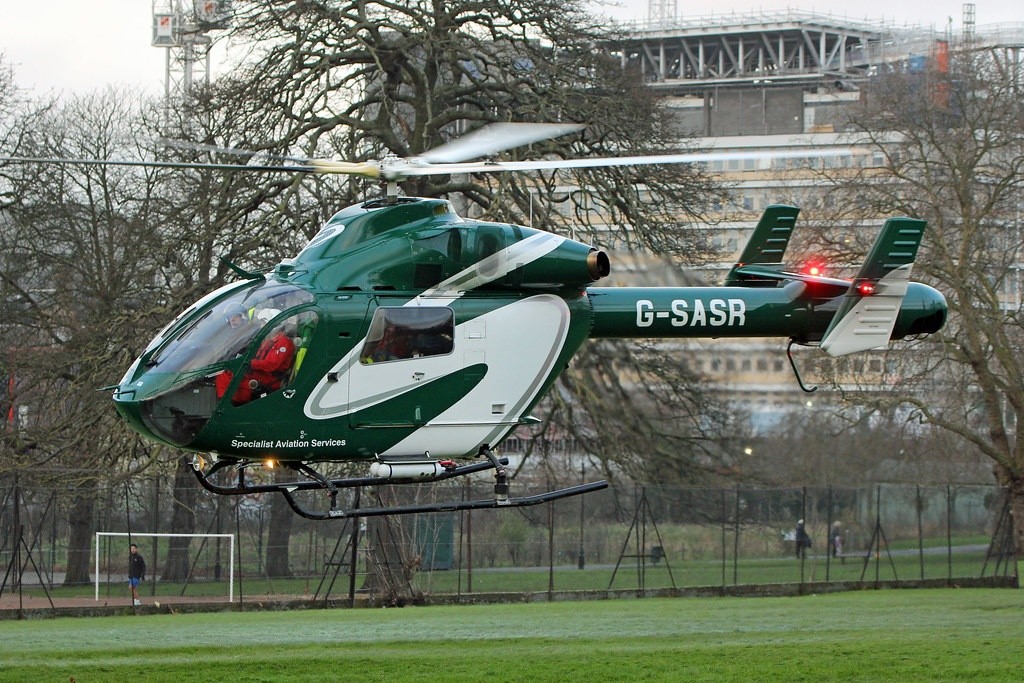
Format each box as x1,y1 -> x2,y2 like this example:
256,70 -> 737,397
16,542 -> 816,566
257,307 -> 287,337
223,300 -> 249,330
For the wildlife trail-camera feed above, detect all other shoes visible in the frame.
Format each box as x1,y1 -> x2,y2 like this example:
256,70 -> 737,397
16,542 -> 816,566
133,599 -> 141,606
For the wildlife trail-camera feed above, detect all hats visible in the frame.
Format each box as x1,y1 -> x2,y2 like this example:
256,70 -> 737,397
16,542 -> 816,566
832,520 -> 842,528
798,520 -> 804,525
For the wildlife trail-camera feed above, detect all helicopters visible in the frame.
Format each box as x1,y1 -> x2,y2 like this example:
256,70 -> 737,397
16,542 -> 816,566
0,121 -> 948,519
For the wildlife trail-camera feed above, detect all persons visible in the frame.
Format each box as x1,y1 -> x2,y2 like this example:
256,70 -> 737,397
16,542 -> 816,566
796,519 -> 811,559
216,309 -> 295,406
368,311 -> 412,362
830,521 -> 842,558
209,301 -> 250,364
128,544 -> 146,605
